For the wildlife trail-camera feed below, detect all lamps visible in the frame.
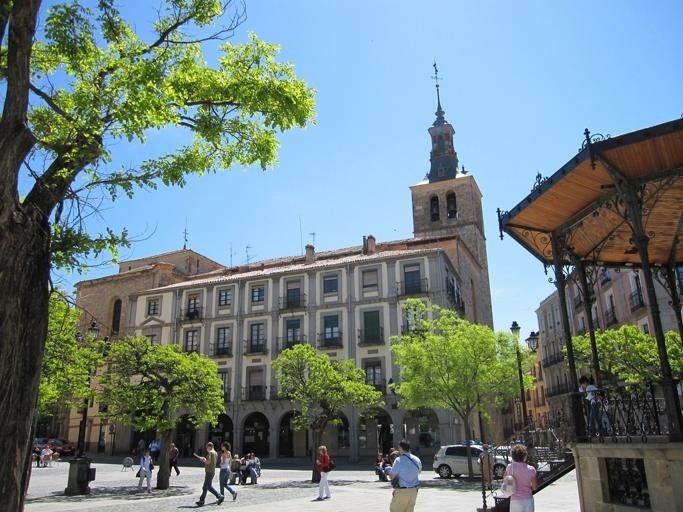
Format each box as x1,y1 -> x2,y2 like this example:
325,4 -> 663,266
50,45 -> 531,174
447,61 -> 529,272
453,416 -> 458,425
360,425 -> 366,431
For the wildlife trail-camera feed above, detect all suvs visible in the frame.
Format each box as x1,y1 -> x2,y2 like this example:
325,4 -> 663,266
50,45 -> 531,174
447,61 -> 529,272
431,439 -> 513,480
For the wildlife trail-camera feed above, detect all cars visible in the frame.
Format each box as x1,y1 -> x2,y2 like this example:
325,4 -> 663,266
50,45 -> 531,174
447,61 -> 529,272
32,436 -> 74,458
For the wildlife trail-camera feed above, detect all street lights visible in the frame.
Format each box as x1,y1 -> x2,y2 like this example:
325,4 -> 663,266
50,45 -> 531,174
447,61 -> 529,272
64,319 -> 110,494
499,319 -> 541,466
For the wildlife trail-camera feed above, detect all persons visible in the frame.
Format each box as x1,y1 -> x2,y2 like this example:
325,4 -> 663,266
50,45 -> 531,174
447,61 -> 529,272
384,439 -> 423,512
232,453 -> 261,485
374,447 -> 399,481
578,375 -> 613,436
133,435 -> 161,465
478,444 -> 494,489
169,443 -> 180,476
502,444 -> 538,512
316,446 -> 330,501
219,442 -> 238,500
139,449 -> 153,493
195,442 -> 224,506
32,445 -> 53,467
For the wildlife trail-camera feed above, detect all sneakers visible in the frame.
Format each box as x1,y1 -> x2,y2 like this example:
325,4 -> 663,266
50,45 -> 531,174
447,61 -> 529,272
195,500 -> 205,507
232,491 -> 237,500
217,495 -> 224,505
228,479 -> 257,486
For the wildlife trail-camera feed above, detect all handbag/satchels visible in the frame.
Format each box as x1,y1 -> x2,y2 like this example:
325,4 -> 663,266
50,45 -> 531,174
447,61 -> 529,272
311,460 -> 321,483
500,463 -> 517,497
149,458 -> 155,471
238,459 -> 246,471
390,473 -> 399,488
322,454 -> 337,473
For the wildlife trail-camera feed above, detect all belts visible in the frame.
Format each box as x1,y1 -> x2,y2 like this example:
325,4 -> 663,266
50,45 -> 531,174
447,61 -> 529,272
397,484 -> 418,489
